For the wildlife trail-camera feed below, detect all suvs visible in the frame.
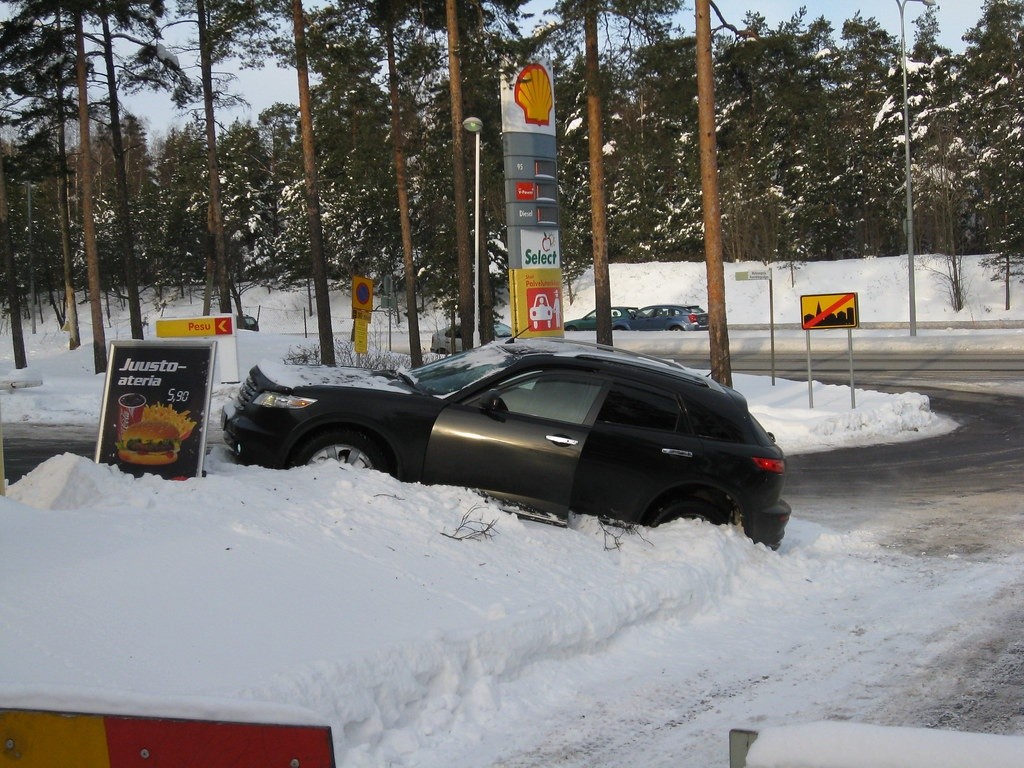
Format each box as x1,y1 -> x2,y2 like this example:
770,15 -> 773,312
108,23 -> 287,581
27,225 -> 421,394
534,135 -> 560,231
215,335 -> 792,554
237,315 -> 260,332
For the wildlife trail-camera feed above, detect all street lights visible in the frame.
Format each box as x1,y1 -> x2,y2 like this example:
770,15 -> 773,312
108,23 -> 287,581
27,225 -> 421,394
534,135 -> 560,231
899,0 -> 938,336
26,168 -> 76,334
462,117 -> 485,352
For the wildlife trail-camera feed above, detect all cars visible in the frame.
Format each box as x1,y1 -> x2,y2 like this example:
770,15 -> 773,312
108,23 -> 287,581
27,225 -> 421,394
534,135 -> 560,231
564,306 -> 645,332
430,321 -> 513,356
612,305 -> 709,333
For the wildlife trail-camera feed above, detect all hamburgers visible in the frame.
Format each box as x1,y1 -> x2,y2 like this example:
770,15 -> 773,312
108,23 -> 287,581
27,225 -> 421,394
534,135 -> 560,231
114,419 -> 182,464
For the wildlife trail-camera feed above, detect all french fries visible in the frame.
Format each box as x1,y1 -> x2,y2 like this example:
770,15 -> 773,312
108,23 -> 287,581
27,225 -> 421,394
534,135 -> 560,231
142,401 -> 197,439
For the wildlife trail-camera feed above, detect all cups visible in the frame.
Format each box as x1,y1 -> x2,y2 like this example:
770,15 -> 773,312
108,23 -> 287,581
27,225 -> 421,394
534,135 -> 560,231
116,393 -> 146,445
